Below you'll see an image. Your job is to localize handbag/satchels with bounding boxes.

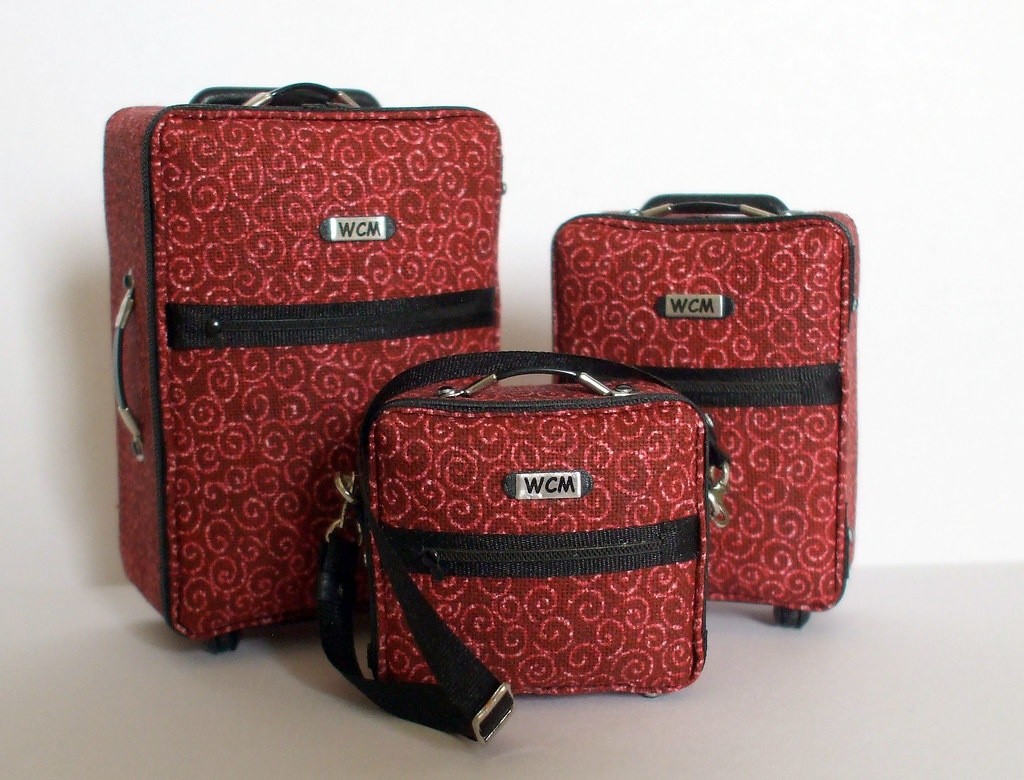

[318,351,710,741]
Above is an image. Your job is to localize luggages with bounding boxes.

[551,194,861,629]
[103,83,507,654]
